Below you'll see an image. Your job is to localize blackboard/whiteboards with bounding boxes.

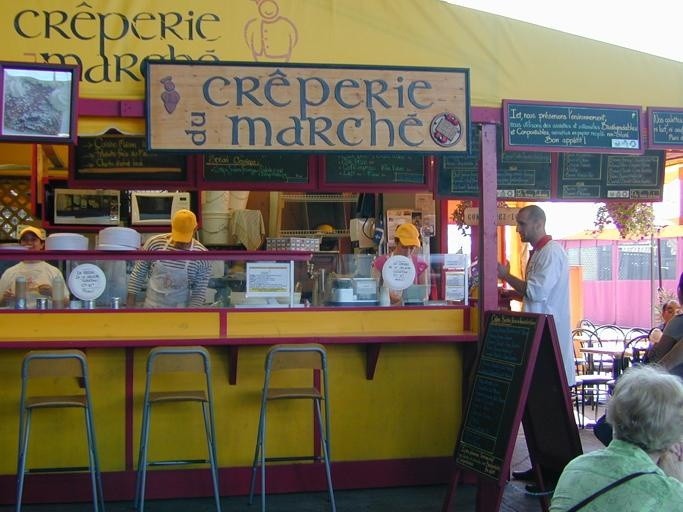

[507,101,646,155]
[647,105,683,150]
[198,131,316,190]
[70,134,197,188]
[443,126,552,201]
[452,309,546,486]
[319,133,433,191]
[555,149,664,202]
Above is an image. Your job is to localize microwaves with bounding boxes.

[52,188,120,225]
[130,191,191,226]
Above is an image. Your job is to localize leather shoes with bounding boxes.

[512,468,534,480]
[525,484,537,492]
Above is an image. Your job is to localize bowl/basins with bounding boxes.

[275,291,301,304]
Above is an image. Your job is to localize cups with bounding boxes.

[35,298,47,310]
[84,300,95,309]
[333,288,353,302]
[69,300,81,309]
[378,286,390,307]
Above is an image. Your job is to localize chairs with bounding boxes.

[244,340,338,511]
[11,346,106,511]
[566,315,657,431]
[130,343,224,511]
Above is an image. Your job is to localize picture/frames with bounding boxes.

[0,58,80,146]
[144,58,472,157]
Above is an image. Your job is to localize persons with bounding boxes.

[648,271,682,371]
[645,300,682,363]
[547,361,683,512]
[370,222,429,305]
[498,204,577,494]
[0,227,71,306]
[126,210,212,308]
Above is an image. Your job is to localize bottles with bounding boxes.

[110,297,119,310]
[51,275,64,309]
[14,276,27,310]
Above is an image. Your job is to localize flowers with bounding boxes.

[593,199,664,245]
[450,194,511,239]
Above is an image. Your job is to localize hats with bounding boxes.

[21,226,42,241]
[394,223,421,248]
[317,224,333,234]
[172,210,197,243]
